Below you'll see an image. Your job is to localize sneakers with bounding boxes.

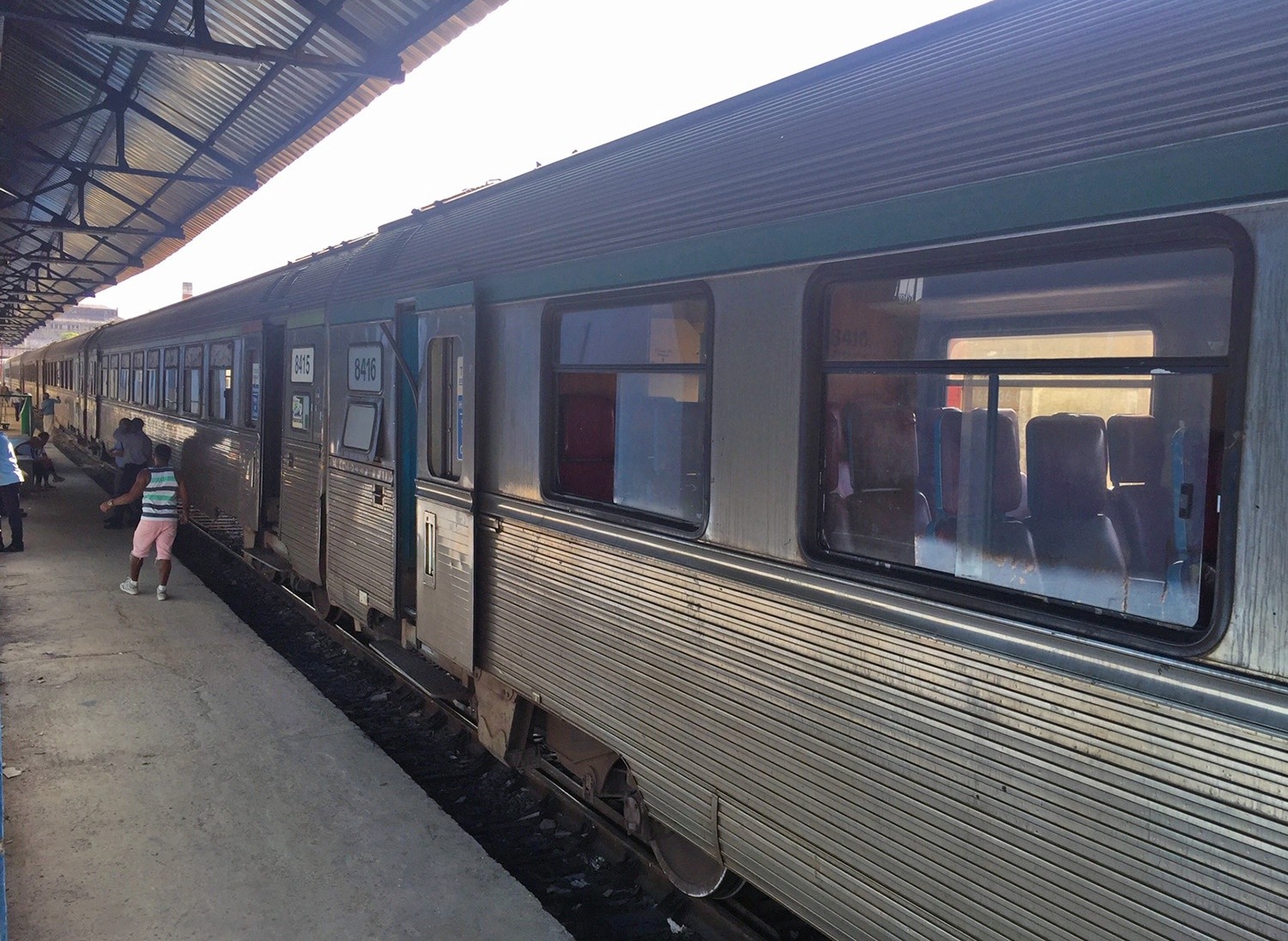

[120,576,138,594]
[156,585,167,600]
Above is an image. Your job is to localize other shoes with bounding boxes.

[0,542,24,551]
[104,517,123,530]
[52,477,65,483]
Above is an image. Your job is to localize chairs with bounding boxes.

[825,391,1195,623]
[563,387,713,510]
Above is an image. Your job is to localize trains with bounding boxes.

[0,0,1288,941]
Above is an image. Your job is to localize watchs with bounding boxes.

[107,499,114,507]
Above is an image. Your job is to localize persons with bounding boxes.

[41,393,61,433]
[99,444,190,601]
[0,382,8,407]
[104,417,154,530]
[10,388,23,421]
[0,432,65,553]
